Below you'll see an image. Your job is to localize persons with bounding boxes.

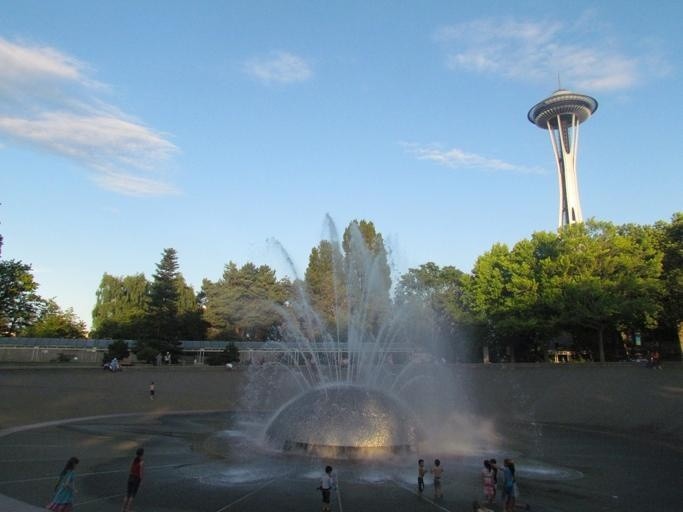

[647,348,665,371]
[163,351,172,367]
[430,458,445,504]
[46,456,80,511]
[155,352,162,367]
[118,448,144,512]
[317,465,336,511]
[108,357,119,373]
[415,459,428,502]
[148,381,157,402]
[480,458,519,511]
